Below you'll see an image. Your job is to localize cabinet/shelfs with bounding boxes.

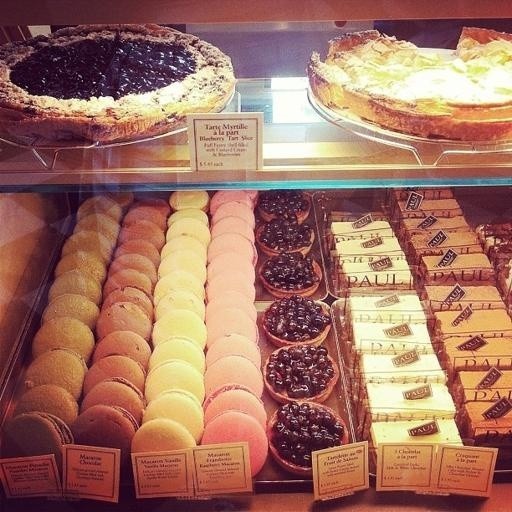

[0,0,512,510]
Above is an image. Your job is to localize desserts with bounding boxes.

[254,187,511,477]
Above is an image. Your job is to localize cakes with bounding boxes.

[0,23,236,141]
[308,26,512,141]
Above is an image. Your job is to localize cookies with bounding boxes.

[5,191,268,478]
